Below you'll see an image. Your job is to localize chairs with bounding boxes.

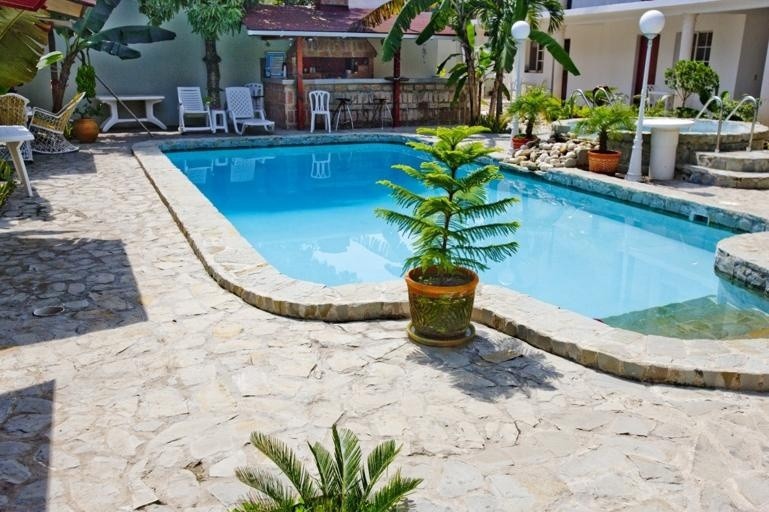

[243,83,266,120]
[224,86,275,135]
[0,92,33,161]
[29,90,85,155]
[176,86,213,135]
[307,90,331,133]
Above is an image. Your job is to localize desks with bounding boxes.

[96,93,167,131]
[0,123,34,200]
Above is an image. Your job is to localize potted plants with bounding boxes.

[369,118,521,349]
[573,100,637,175]
[503,86,558,152]
[72,105,99,144]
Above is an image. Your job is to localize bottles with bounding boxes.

[281,63,287,79]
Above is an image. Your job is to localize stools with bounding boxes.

[332,97,354,131]
[211,109,228,135]
[371,98,393,130]
